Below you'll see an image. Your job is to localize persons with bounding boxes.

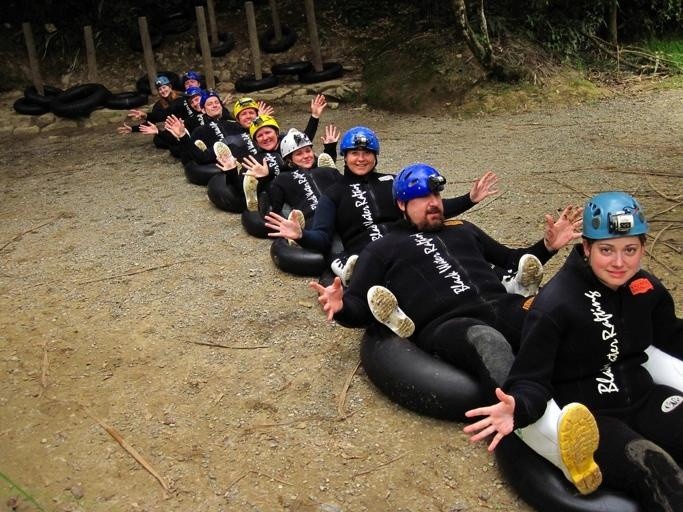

[241,128,359,288]
[215,115,340,250]
[128,76,183,123]
[164,86,265,151]
[165,101,266,165]
[164,94,339,213]
[139,87,211,152]
[463,190,683,512]
[115,70,202,138]
[264,126,544,339]
[308,164,683,496]
[164,93,275,160]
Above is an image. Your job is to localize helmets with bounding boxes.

[582,192,650,240]
[280,128,314,159]
[155,76,171,88]
[249,114,281,141]
[340,127,380,156]
[392,164,447,206]
[200,91,220,110]
[233,97,260,123]
[182,71,200,84]
[185,87,203,101]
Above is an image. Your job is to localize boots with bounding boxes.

[286,209,305,247]
[501,254,543,298]
[645,345,683,391]
[243,170,259,211]
[514,397,604,496]
[366,284,415,338]
[213,141,241,174]
[317,153,336,168]
[195,140,207,152]
[330,254,360,288]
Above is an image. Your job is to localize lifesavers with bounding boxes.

[492,426,657,512]
[104,91,148,110]
[168,142,183,156]
[240,192,282,239]
[270,238,326,277]
[186,159,223,186]
[23,85,63,105]
[316,264,346,308]
[235,72,277,93]
[14,97,51,116]
[195,30,235,56]
[259,24,297,53]
[207,173,246,214]
[271,61,312,76]
[153,135,170,149]
[129,27,164,51]
[298,62,344,83]
[136,71,179,95]
[52,83,111,118]
[180,150,193,165]
[176,74,218,92]
[359,323,499,424]
